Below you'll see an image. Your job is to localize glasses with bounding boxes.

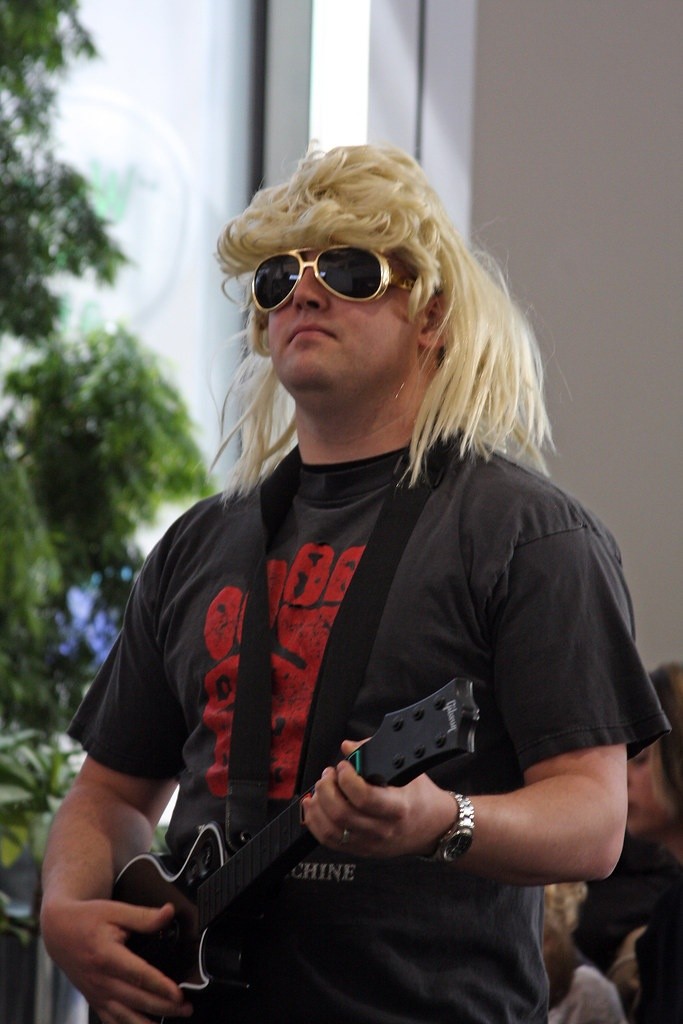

[251,244,416,313]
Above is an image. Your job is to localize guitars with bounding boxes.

[112,676,480,1008]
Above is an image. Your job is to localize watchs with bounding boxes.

[419,791,474,869]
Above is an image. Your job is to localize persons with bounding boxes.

[541,881,623,1024]
[626,661,683,914]
[38,143,662,1024]
